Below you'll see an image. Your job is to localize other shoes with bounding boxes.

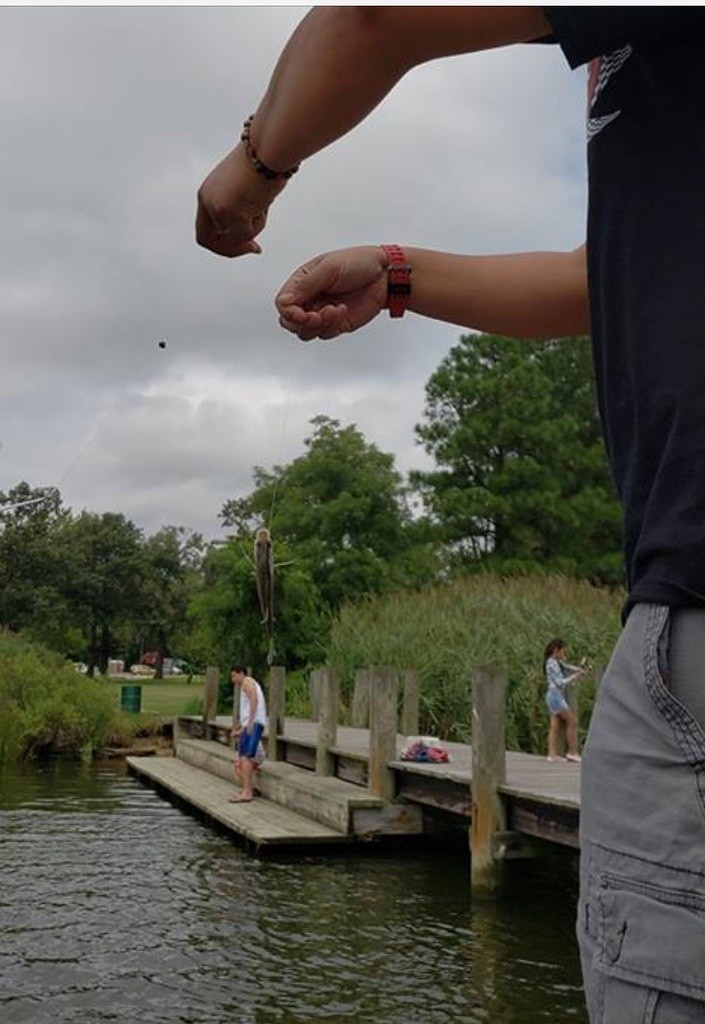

[567,754,581,762]
[547,756,568,762]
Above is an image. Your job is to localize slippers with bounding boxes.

[230,800,250,802]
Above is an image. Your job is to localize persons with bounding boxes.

[229,665,266,803]
[195,6,705,1024]
[543,639,584,763]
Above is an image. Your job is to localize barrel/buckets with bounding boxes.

[121,685,141,713]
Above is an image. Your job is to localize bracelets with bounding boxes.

[381,243,412,319]
[240,114,301,181]
